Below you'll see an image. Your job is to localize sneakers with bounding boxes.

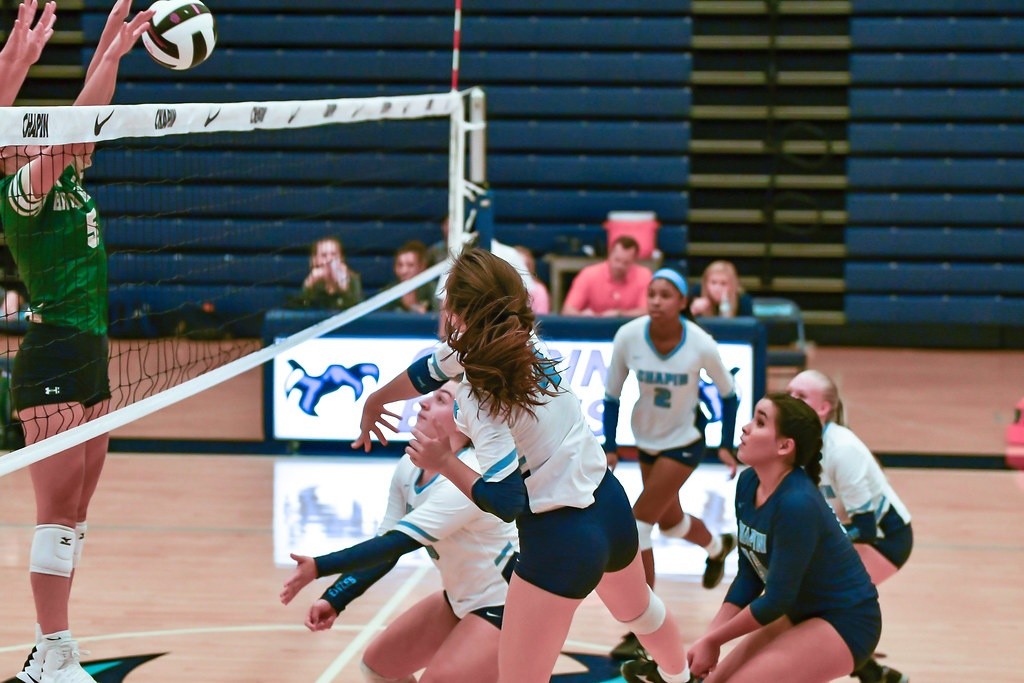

[879,666,909,682]
[703,533,736,588]
[40,641,98,683]
[15,637,64,683]
[611,631,643,660]
[621,659,697,683]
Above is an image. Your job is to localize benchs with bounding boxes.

[0,0,1024,345]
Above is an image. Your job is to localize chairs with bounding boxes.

[750,297,807,377]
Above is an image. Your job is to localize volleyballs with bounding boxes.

[141,0,218,71]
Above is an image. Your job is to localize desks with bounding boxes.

[258,308,765,466]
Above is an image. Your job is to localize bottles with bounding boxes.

[719,288,732,318]
[330,261,350,284]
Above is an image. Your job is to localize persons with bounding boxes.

[281,216,913,683]
[0,0,157,683]
